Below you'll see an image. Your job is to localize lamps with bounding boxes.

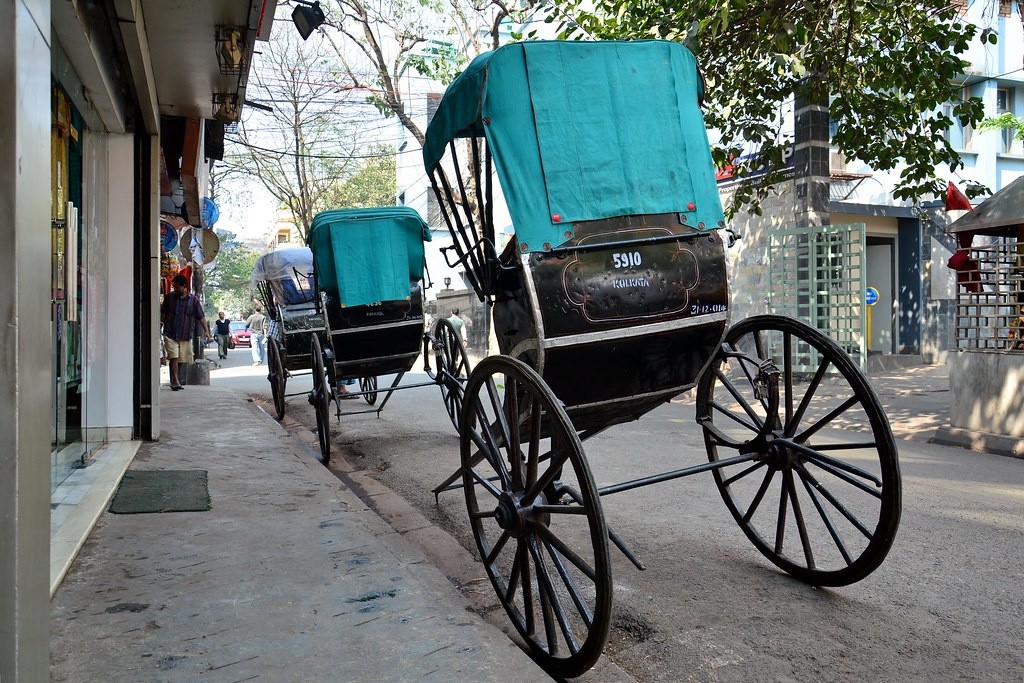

[214,97,235,126]
[221,29,241,71]
[291,0,325,40]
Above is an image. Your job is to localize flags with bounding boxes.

[946,182,975,216]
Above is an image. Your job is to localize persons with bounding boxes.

[337,378,360,399]
[215,311,230,360]
[159,275,211,391]
[244,306,268,366]
[444,308,468,366]
[419,308,436,355]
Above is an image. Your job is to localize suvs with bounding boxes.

[214,321,252,349]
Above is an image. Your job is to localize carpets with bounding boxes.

[109,470,212,514]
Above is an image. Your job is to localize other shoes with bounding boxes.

[220,356,223,359]
[252,361,261,366]
[223,357,226,359]
[170,383,184,391]
[341,391,360,399]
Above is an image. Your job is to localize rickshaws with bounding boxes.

[308,207,472,463]
[432,40,903,678]
[252,247,328,419]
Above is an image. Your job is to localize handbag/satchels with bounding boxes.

[227,337,235,349]
